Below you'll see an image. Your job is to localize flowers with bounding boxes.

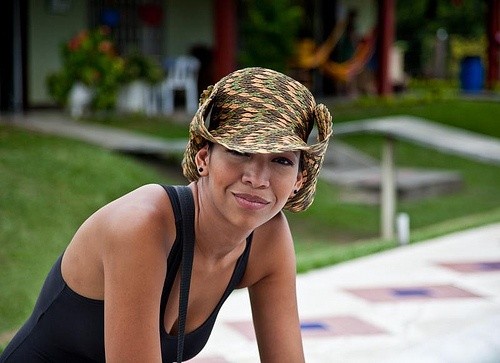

[42,26,164,92]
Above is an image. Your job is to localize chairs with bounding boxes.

[150,57,199,119]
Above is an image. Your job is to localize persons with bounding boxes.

[0,67,333,363]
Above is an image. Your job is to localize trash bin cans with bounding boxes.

[461,54,486,93]
